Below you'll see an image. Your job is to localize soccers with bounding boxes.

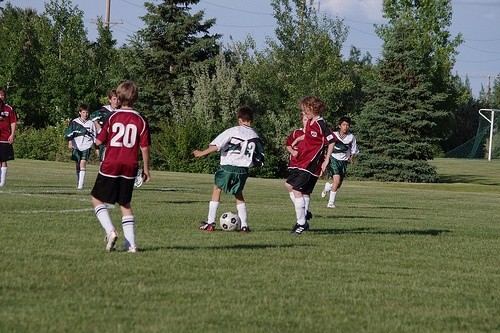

[219,212,240,232]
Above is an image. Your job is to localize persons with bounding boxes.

[65,103,101,190]
[0,86,18,187]
[90,80,151,253]
[89,89,120,165]
[192,106,265,234]
[321,116,359,208]
[285,110,313,221]
[285,95,338,234]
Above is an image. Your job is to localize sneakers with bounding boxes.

[235,226,249,231]
[327,203,336,208]
[305,210,312,220]
[104,230,118,252]
[198,221,216,231]
[290,220,309,235]
[322,181,331,198]
[118,246,138,252]
